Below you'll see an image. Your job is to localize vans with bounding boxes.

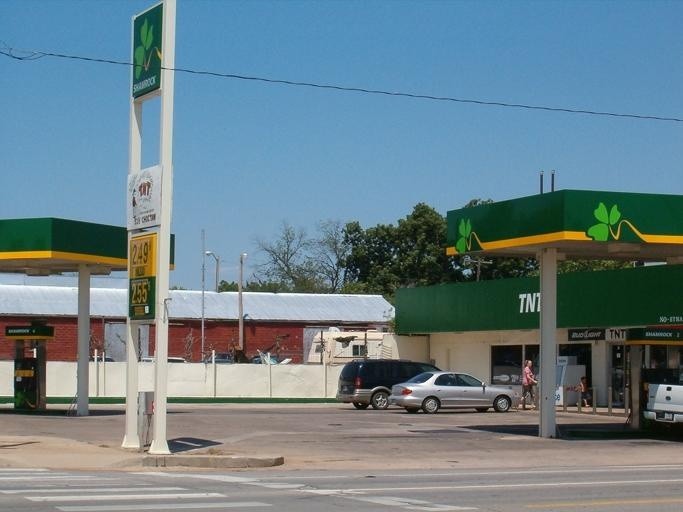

[336,360,442,410]
[307,331,395,365]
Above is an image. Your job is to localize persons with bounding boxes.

[515,360,538,411]
[574,376,591,407]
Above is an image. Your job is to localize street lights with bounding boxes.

[205,250,253,350]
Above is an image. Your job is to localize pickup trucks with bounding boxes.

[643,383,683,424]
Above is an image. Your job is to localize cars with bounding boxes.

[387,370,520,412]
[86,353,280,364]
